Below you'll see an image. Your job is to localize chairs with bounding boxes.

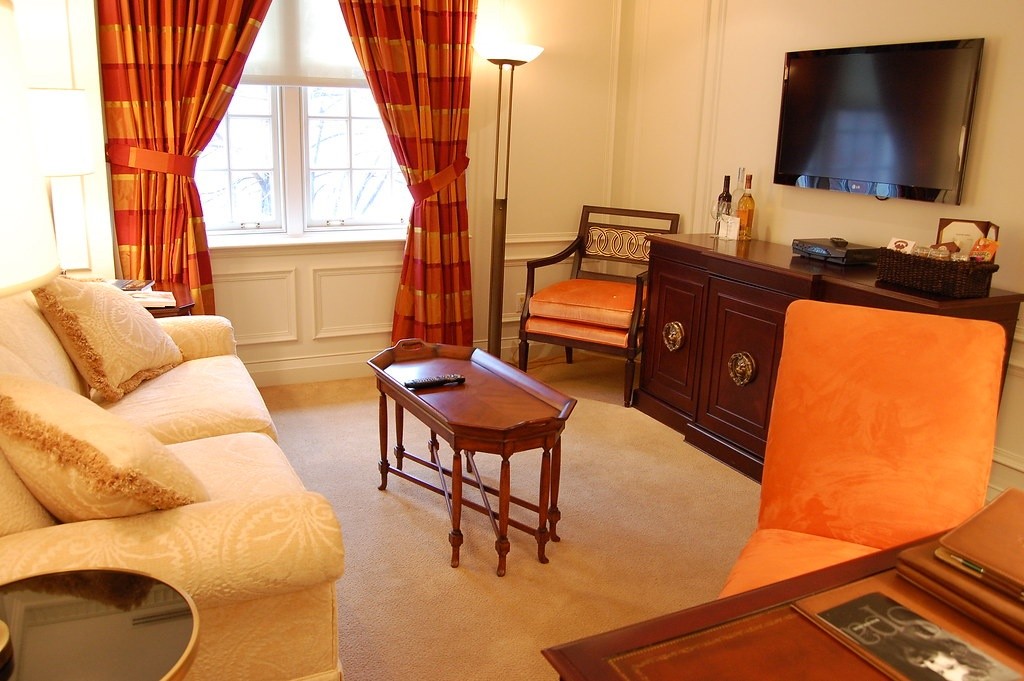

[518,203,680,409]
[716,296,1008,598]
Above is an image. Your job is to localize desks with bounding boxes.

[364,337,579,577]
[541,521,1022,681]
[0,567,200,681]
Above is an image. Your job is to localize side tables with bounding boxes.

[103,279,196,318]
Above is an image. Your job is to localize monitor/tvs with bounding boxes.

[773,38,984,205]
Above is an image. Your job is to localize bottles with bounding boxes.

[737,174,755,241]
[715,175,732,235]
[731,167,745,218]
[911,246,970,262]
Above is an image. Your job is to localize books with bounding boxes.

[895,489,1024,649]
[112,280,177,306]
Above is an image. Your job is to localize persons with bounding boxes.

[921,639,994,681]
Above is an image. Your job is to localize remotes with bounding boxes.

[830,238,848,247]
[404,374,465,388]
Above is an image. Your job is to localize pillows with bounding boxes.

[32,275,186,403]
[0,370,211,524]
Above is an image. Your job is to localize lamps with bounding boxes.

[475,38,546,365]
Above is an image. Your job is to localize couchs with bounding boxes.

[0,264,346,681]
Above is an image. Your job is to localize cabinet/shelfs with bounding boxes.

[631,237,1024,489]
